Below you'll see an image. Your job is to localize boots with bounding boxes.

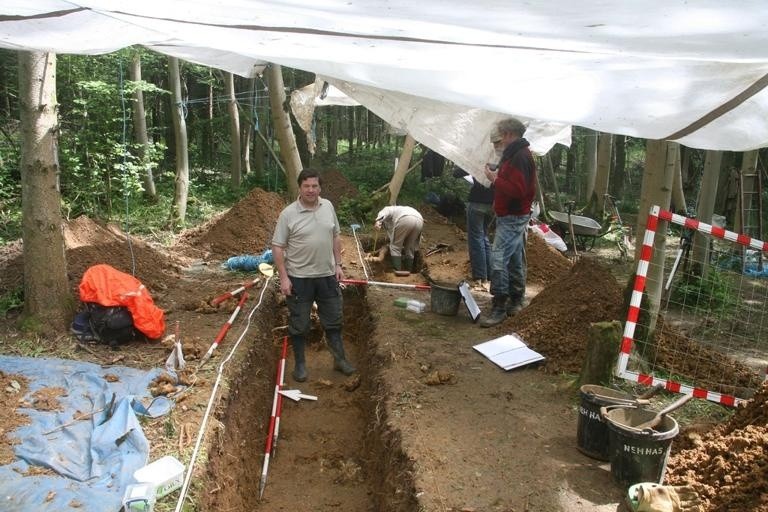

[392,256,403,271]
[404,259,416,272]
[293,336,308,382]
[325,331,360,376]
[507,292,522,316]
[480,296,507,327]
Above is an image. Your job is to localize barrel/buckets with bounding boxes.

[429,281,461,317]
[600,403,680,491]
[575,383,640,462]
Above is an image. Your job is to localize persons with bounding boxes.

[375,205,424,273]
[479,118,538,329]
[451,168,494,281]
[489,126,536,298]
[272,168,357,381]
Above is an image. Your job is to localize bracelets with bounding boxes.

[335,261,342,266]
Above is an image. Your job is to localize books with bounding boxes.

[472,334,545,370]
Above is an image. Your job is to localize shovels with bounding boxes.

[424,244,450,257]
[660,236,690,312]
[278,390,317,403]
[634,394,694,430]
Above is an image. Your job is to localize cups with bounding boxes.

[487,158,496,171]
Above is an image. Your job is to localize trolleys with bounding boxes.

[548,192,632,251]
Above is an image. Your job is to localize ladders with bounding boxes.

[739,170,763,275]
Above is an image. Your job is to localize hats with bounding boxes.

[489,126,504,142]
